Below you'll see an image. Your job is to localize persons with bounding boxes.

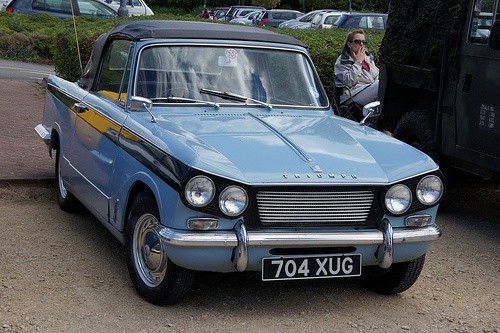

[334,29,379,106]
[202,9,210,18]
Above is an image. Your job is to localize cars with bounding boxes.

[78,0,156,18]
[195,5,266,26]
[311,10,355,32]
[331,12,388,46]
[279,9,328,31]
[6,0,123,22]
[228,10,262,26]
[252,8,306,29]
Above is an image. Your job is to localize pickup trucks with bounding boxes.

[33,18,448,307]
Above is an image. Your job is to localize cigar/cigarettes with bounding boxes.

[361,46,363,50]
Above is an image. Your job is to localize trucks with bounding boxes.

[375,0,500,187]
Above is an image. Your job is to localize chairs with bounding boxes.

[333,77,362,123]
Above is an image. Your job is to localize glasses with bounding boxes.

[348,39,367,45]
